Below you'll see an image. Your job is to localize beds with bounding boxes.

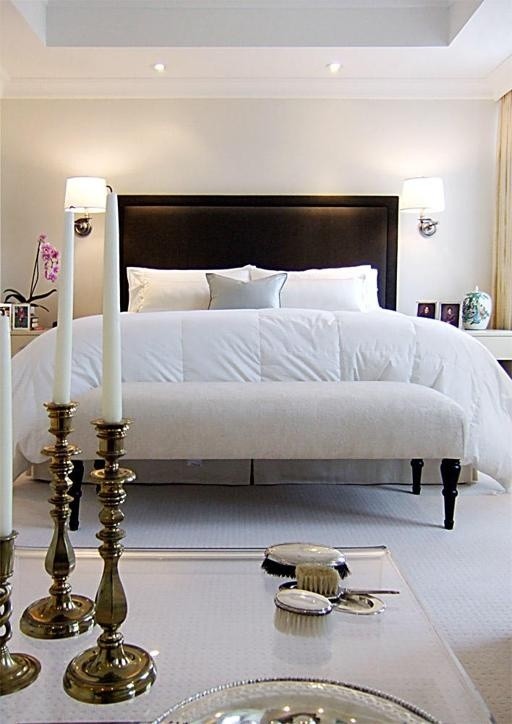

[10,191,511,530]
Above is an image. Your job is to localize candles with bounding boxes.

[0,309,14,539]
[52,202,82,405]
[97,182,125,426]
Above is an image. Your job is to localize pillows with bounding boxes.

[124,261,258,315]
[203,268,288,311]
[247,263,379,313]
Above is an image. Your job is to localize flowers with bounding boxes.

[1,229,61,307]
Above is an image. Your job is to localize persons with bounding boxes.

[17,307,26,327]
[446,307,457,325]
[420,306,432,318]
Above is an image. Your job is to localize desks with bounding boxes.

[0,541,498,724]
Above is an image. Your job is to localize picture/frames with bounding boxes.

[12,301,30,331]
[415,296,439,322]
[0,301,13,330]
[437,298,461,329]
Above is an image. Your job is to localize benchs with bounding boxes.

[16,373,473,537]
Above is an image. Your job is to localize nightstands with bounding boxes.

[455,327,512,367]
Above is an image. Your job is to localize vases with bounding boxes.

[27,304,40,329]
[459,282,493,330]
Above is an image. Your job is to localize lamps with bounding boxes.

[396,175,447,235]
[60,175,114,237]
